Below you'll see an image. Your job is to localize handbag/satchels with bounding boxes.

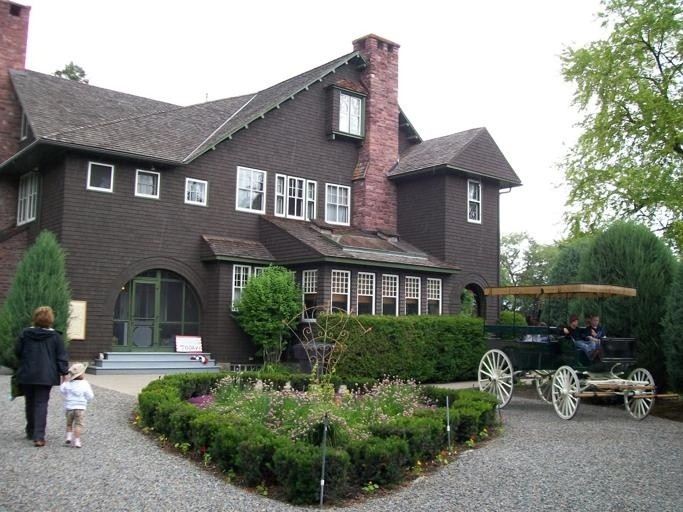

[11,374,25,397]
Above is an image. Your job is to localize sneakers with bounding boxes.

[64,437,72,444]
[72,439,81,447]
[34,440,45,446]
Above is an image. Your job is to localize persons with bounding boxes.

[537,320,553,344]
[521,314,539,342]
[13,304,68,447]
[59,361,94,450]
[556,314,601,363]
[586,312,607,348]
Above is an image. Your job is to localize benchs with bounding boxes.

[557,325,600,367]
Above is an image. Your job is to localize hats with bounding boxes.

[68,362,89,381]
[570,314,578,323]
